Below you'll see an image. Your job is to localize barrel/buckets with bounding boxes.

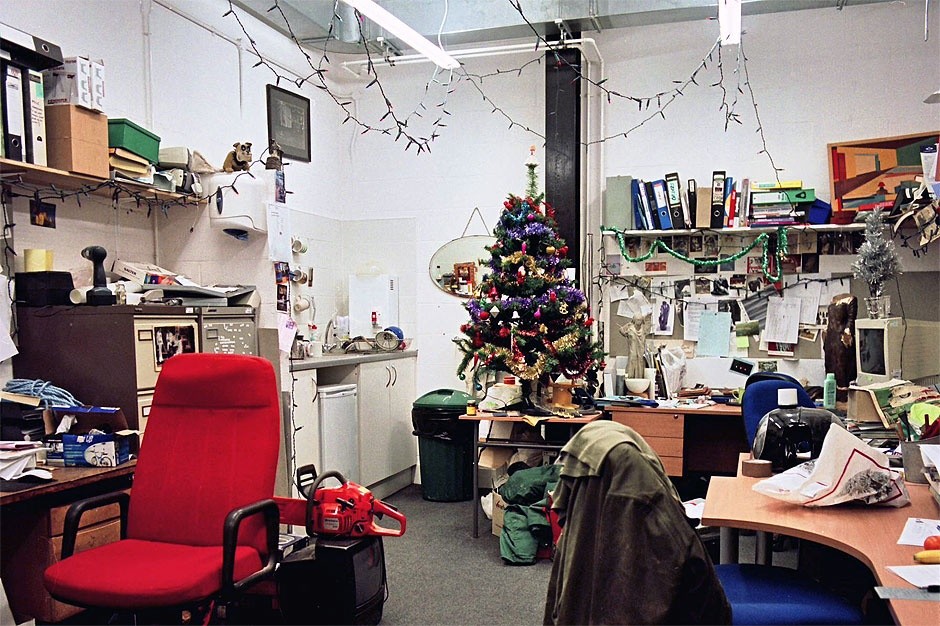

[900,435,939,484]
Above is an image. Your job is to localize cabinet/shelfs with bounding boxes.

[324,355,419,490]
[283,367,321,541]
[0,488,139,626]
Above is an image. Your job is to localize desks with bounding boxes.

[460,407,602,549]
[603,396,847,482]
[0,452,140,626]
[700,473,940,626]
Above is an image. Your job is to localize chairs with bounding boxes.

[748,406,846,474]
[553,413,865,626]
[745,371,803,386]
[44,352,285,626]
[740,377,821,449]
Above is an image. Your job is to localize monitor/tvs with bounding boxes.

[854,318,940,387]
[275,535,386,626]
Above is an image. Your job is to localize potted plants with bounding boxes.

[849,205,902,321]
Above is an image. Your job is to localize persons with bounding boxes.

[705,237,717,256]
[619,314,648,379]
[660,301,669,330]
[627,239,639,258]
[674,237,688,257]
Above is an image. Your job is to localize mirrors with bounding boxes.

[429,234,499,298]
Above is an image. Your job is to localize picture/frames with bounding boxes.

[266,82,312,164]
[826,129,940,214]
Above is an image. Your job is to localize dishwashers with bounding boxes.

[317,383,360,490]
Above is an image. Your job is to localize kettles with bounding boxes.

[289,336,305,360]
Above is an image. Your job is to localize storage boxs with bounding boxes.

[113,258,177,286]
[477,449,510,490]
[846,391,881,420]
[798,198,829,223]
[40,409,64,463]
[89,59,107,112]
[605,175,633,230]
[48,140,110,180]
[45,54,91,109]
[491,489,508,537]
[488,420,518,440]
[45,105,109,145]
[695,187,712,228]
[64,402,136,472]
[108,117,161,163]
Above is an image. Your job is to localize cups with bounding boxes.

[307,341,322,357]
[292,265,308,284]
[294,294,311,311]
[291,236,307,253]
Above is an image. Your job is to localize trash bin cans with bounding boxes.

[412,389,481,501]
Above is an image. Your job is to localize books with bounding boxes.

[723,176,804,227]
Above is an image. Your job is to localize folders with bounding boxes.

[651,179,673,229]
[711,171,725,228]
[638,183,654,230]
[749,187,815,202]
[665,173,684,229]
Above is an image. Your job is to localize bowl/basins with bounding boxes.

[399,338,412,350]
[375,329,400,349]
[625,378,650,393]
[354,341,374,351]
[384,326,403,342]
[742,459,772,477]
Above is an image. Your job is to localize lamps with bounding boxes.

[338,0,462,69]
[718,0,742,47]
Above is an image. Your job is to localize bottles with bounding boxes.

[466,400,477,416]
[823,373,836,409]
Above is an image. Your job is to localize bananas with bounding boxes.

[913,550,940,564]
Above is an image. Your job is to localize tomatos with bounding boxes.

[924,535,940,550]
